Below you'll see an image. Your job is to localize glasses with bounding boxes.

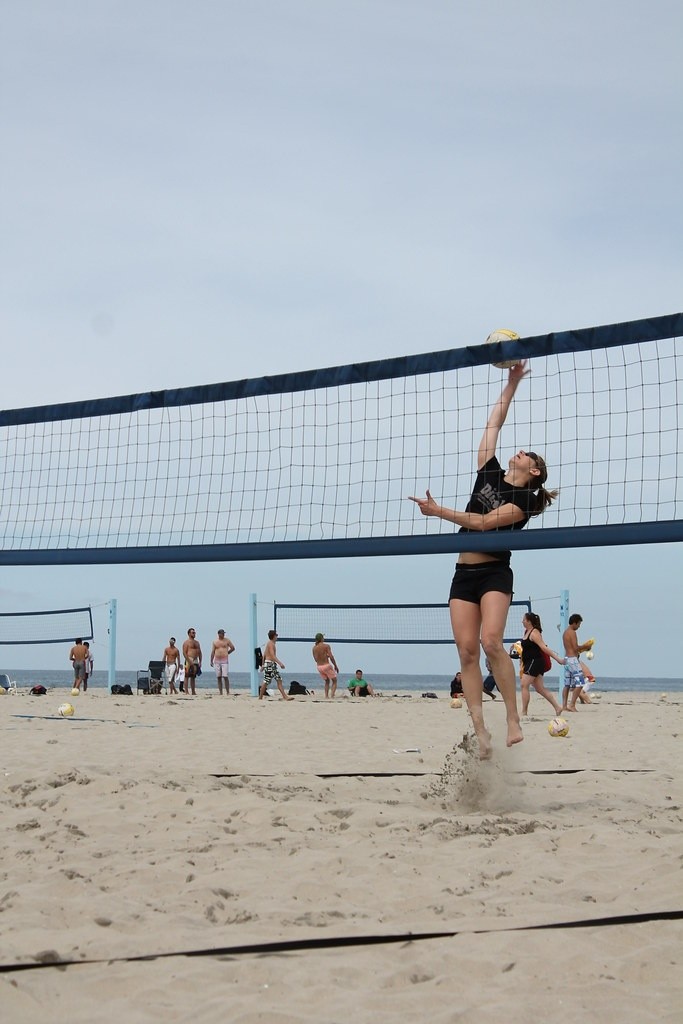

[526,452,539,470]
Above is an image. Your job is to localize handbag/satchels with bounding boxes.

[541,644,551,672]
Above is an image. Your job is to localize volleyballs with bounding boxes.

[0,688,4,694]
[58,703,74,716]
[589,693,602,699]
[71,688,79,696]
[548,718,570,737]
[662,694,667,698]
[586,652,594,660]
[450,699,462,708]
[486,327,523,369]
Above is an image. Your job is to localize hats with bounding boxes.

[218,629,226,633]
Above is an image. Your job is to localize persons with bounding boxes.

[450,672,465,698]
[313,633,339,698]
[511,612,567,716]
[483,658,500,700]
[163,638,180,694]
[562,613,595,711]
[259,630,295,700]
[183,628,202,695]
[210,629,235,695]
[178,664,185,692]
[409,360,558,760]
[70,638,94,691]
[347,670,376,697]
